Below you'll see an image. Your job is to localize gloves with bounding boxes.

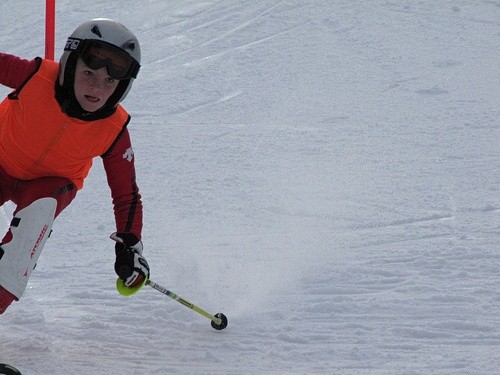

[110,231,150,289]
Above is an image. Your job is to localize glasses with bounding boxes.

[64,37,141,79]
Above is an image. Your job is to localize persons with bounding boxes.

[1,16,151,375]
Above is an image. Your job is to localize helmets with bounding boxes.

[54,17,142,121]
